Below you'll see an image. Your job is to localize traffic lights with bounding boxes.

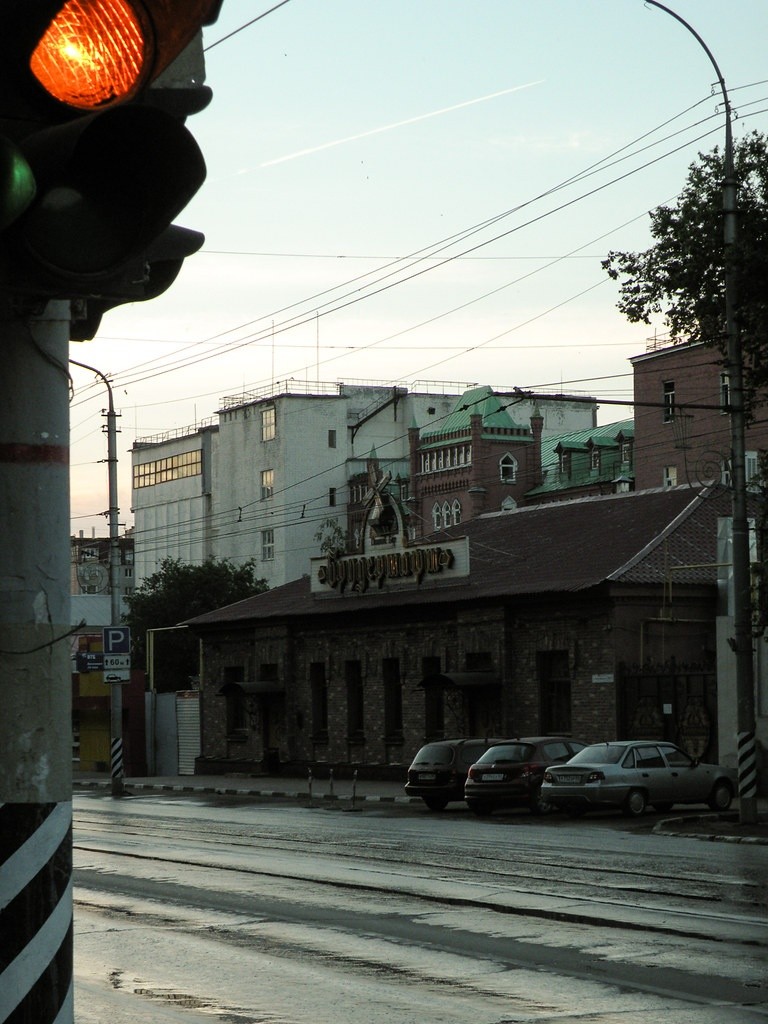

[0,1,224,297]
[73,0,224,354]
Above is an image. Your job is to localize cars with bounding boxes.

[463,736,617,818]
[540,741,738,817]
[403,736,507,813]
